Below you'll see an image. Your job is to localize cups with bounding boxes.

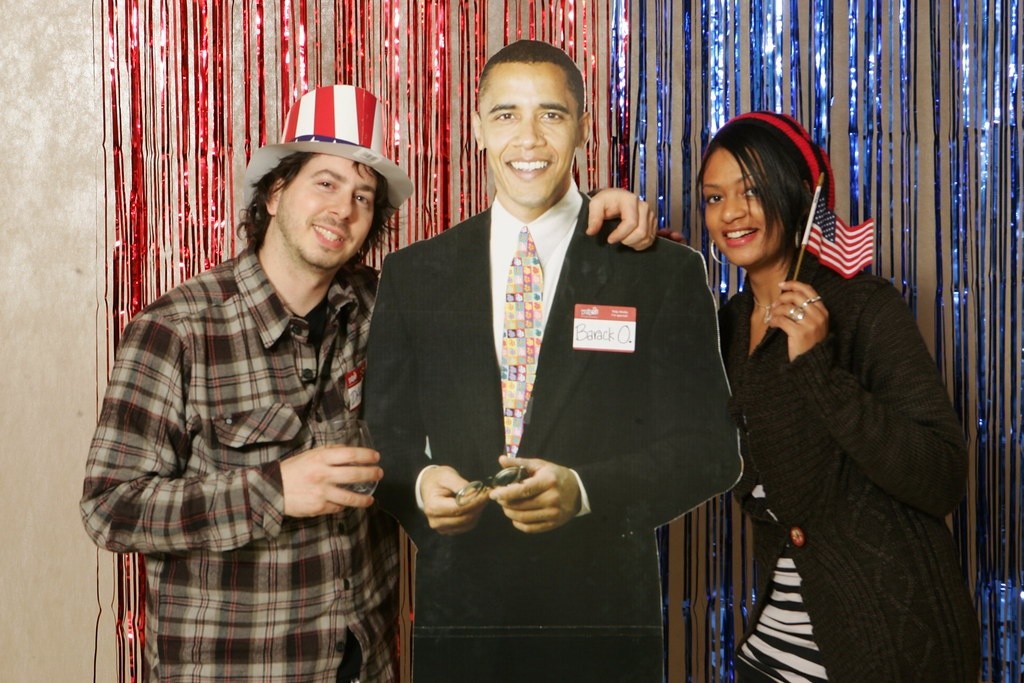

[324,419,379,496]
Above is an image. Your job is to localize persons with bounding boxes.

[658,112,983,683]
[79,84,656,683]
[360,38,745,682]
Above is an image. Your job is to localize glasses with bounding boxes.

[456,465,524,507]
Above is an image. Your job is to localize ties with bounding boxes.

[501,226,545,459]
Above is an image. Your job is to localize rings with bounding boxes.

[801,298,811,308]
[789,305,804,322]
[811,295,822,303]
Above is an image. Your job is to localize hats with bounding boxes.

[245,84,414,226]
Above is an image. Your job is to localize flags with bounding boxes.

[805,194,874,279]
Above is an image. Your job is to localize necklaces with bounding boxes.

[753,296,778,325]
